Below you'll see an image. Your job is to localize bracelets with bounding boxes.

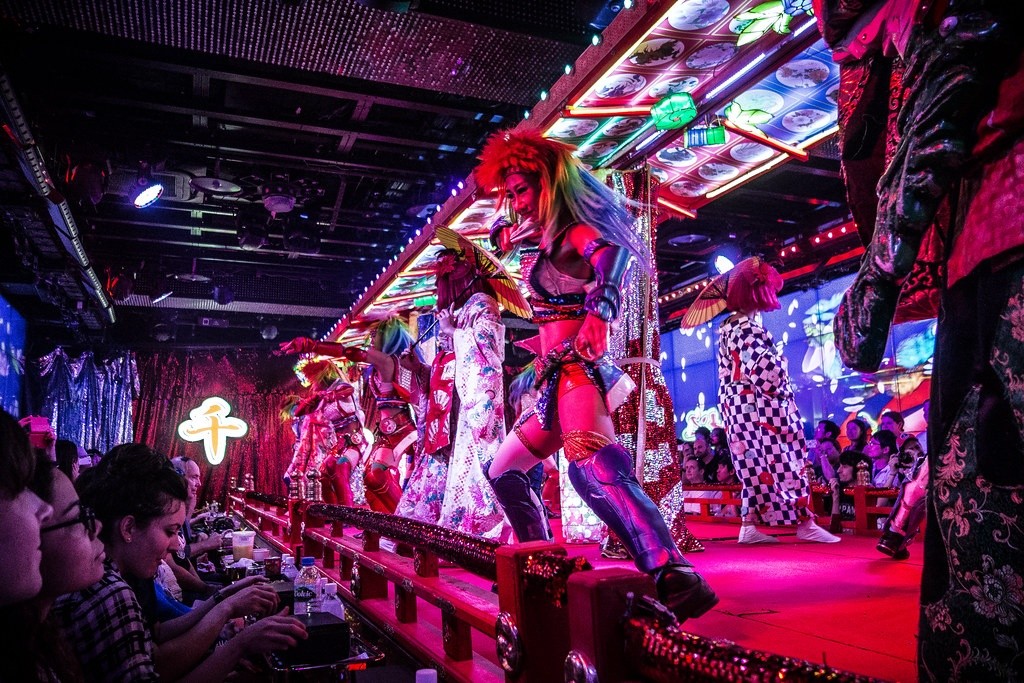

[213,591,224,603]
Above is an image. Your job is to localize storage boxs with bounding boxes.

[277,611,352,662]
[257,581,323,621]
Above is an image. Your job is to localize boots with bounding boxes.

[568,445,718,623]
[482,466,554,592]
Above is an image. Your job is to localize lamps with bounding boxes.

[124,159,171,208]
[144,271,174,306]
[650,89,698,133]
[212,282,233,305]
[712,245,743,274]
[683,118,724,149]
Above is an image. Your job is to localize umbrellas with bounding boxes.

[680,256,759,329]
[401,224,533,358]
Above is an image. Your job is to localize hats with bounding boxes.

[897,432,918,452]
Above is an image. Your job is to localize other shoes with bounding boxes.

[877,533,910,559]
[737,526,777,544]
[796,521,841,543]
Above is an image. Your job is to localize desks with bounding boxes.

[189,512,449,683]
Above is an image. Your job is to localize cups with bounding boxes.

[230,531,256,563]
[245,566,266,579]
[253,550,269,563]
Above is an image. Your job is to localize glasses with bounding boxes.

[869,441,881,446]
[40,506,97,533]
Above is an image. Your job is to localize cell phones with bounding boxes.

[678,445,684,451]
[805,440,817,448]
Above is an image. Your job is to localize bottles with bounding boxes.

[279,554,291,575]
[284,557,298,582]
[293,556,322,618]
[319,578,344,620]
[416,669,437,683]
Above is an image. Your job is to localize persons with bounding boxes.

[676,425,743,518]
[716,260,842,543]
[808,398,930,558]
[0,403,310,683]
[281,129,722,628]
[808,0,1023,683]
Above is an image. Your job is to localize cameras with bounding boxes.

[893,452,912,469]
